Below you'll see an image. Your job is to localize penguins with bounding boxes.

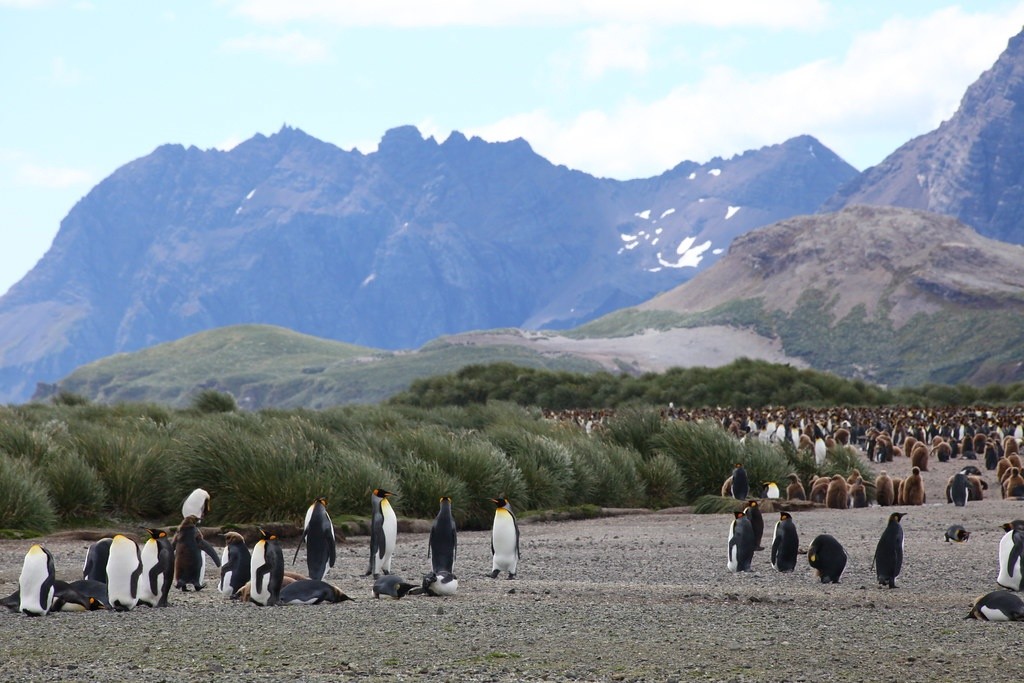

[726,511,755,574]
[742,500,765,551]
[371,574,418,601]
[170,514,222,593]
[49,578,112,614]
[944,525,971,543]
[962,591,1024,622]
[82,537,115,607]
[174,487,210,528]
[870,512,908,589]
[1,543,55,617]
[134,526,175,608]
[426,496,458,578]
[769,511,799,573]
[275,579,357,606]
[538,401,1024,509]
[485,497,521,580]
[409,571,459,597]
[250,526,284,608]
[807,534,848,585]
[996,519,1024,591]
[105,534,144,613]
[218,531,252,600]
[363,489,398,583]
[292,496,336,582]
